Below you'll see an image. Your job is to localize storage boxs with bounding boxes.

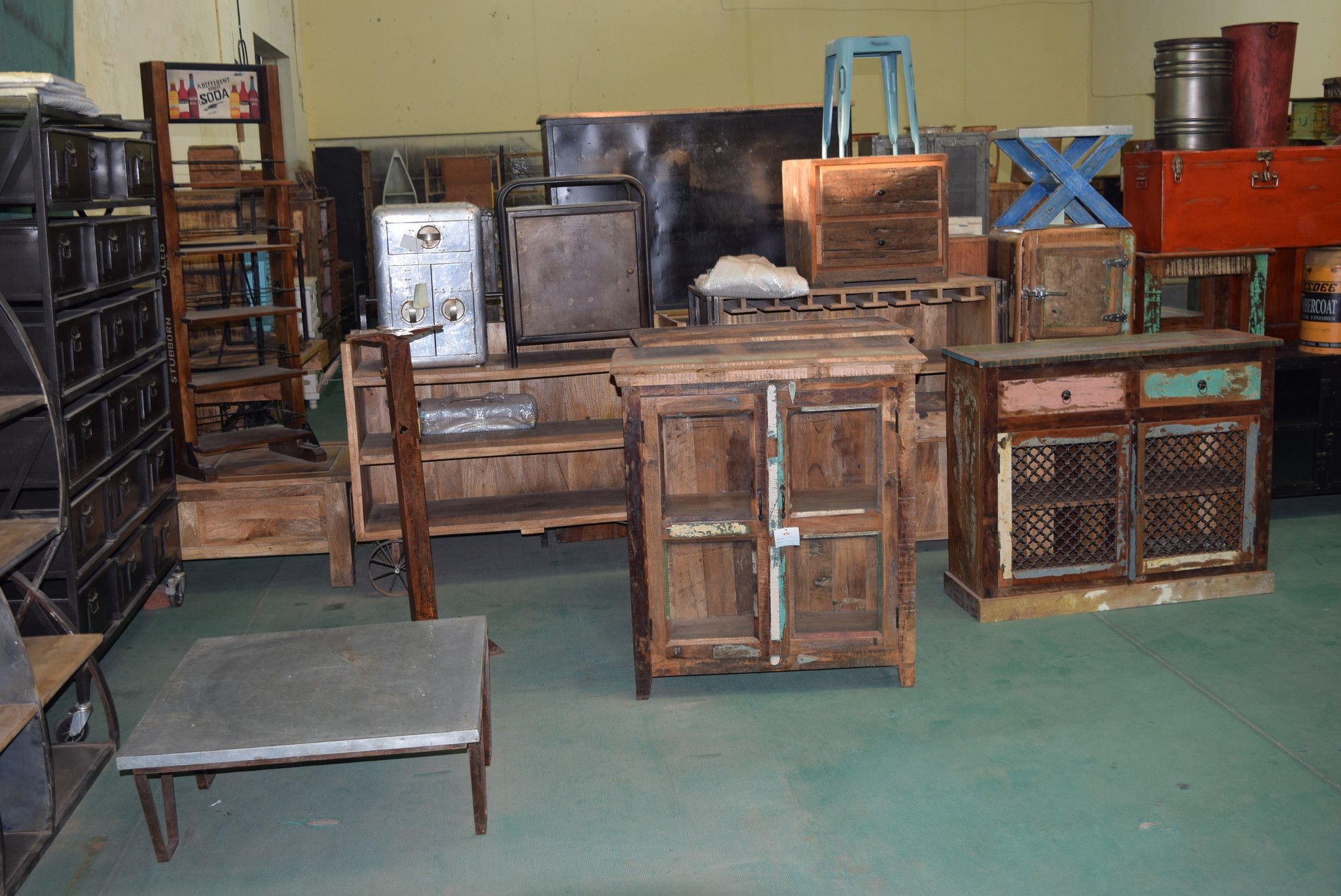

[1121,145,1341,256]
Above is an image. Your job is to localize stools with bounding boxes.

[989,124,1133,229]
[821,35,920,160]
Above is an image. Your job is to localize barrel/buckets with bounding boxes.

[1152,37,1235,150]
[1221,21,1300,147]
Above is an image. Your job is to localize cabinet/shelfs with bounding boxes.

[0,49,1278,896]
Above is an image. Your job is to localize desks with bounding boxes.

[116,616,494,865]
